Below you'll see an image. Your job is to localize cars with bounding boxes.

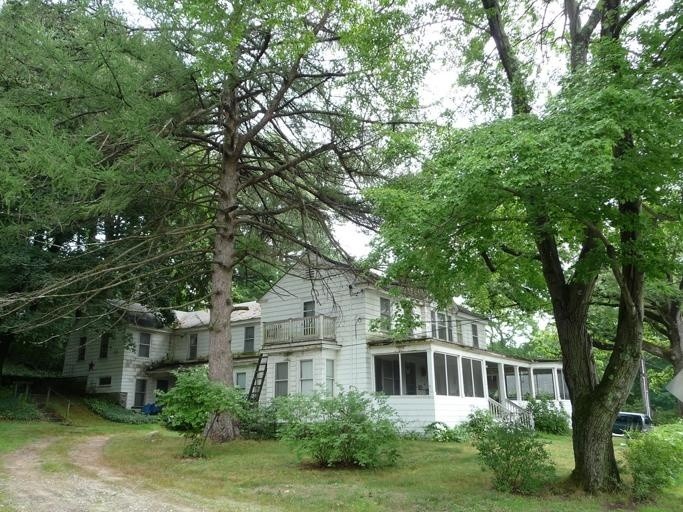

[611,412,654,437]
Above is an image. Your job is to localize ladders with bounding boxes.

[247,354,268,402]
